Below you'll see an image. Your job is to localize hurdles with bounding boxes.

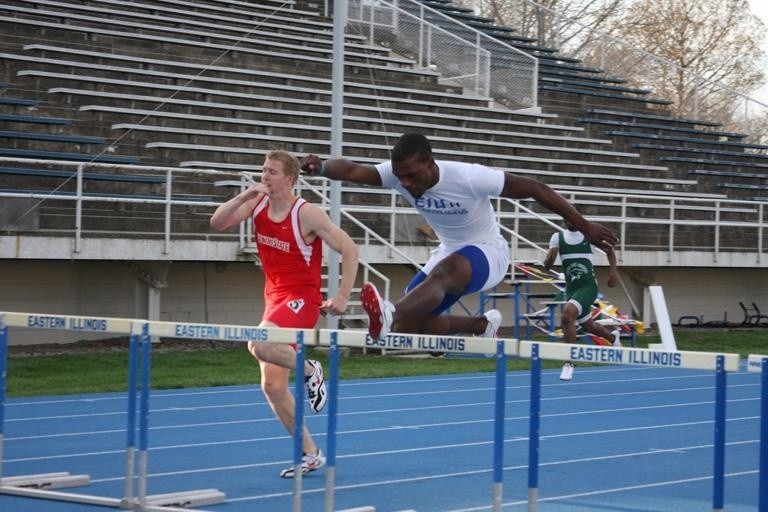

[0,312,767,511]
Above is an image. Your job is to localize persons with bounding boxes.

[538,205,622,380]
[210,150,359,478]
[298,133,619,357]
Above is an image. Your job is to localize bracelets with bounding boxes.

[319,162,325,177]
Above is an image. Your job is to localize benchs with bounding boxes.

[0,0,768,251]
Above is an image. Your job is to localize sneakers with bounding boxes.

[305,359,327,413]
[611,329,622,347]
[560,365,575,381]
[280,447,327,479]
[473,309,502,358]
[361,283,395,339]
